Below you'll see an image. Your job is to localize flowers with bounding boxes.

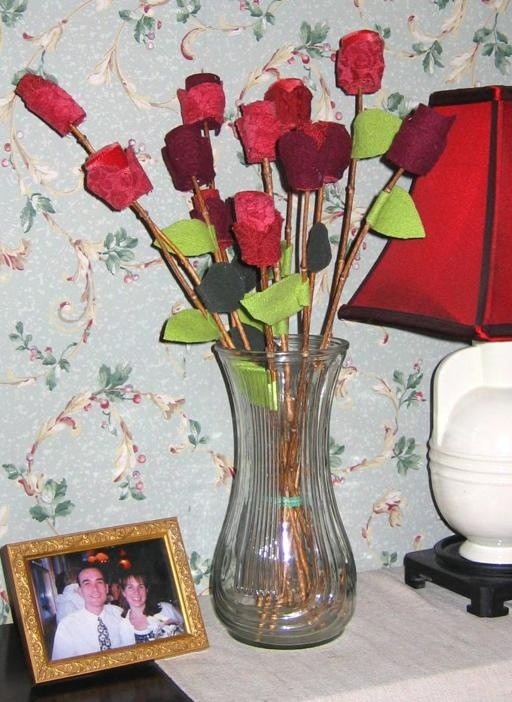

[11,31,461,638]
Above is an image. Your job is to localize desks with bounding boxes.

[2,562,510,701]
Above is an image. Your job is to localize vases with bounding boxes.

[210,334,357,649]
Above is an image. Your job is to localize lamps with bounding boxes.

[335,83,512,619]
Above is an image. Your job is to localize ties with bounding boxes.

[95,616,112,651]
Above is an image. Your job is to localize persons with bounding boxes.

[115,568,184,644]
[52,561,136,664]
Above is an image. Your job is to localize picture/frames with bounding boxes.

[1,516,210,684]
